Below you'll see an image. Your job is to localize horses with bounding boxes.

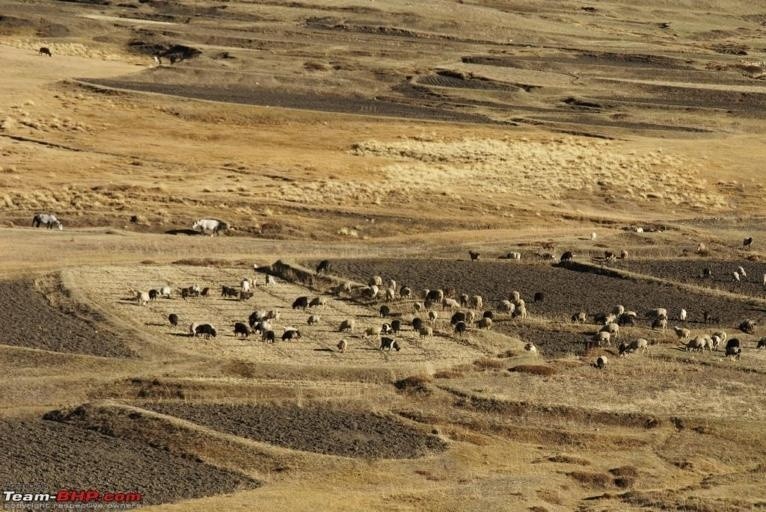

[193,219,220,237]
[31,214,63,230]
[39,48,52,57]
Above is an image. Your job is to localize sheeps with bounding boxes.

[128,275,544,353]
[571,304,766,369]
